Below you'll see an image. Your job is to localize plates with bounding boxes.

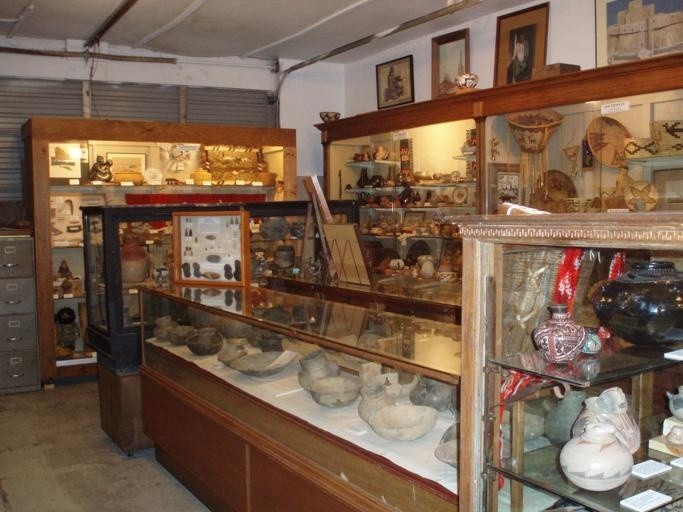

[453,185,469,204]
[623,181,659,213]
[587,117,633,168]
[528,170,577,215]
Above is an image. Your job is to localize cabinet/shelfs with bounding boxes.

[0,237,41,395]
[20,52,682,511]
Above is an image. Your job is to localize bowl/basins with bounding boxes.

[506,108,565,154]
[455,73,478,89]
[436,272,457,283]
[320,112,340,122]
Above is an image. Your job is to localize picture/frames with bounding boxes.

[374,0,550,112]
